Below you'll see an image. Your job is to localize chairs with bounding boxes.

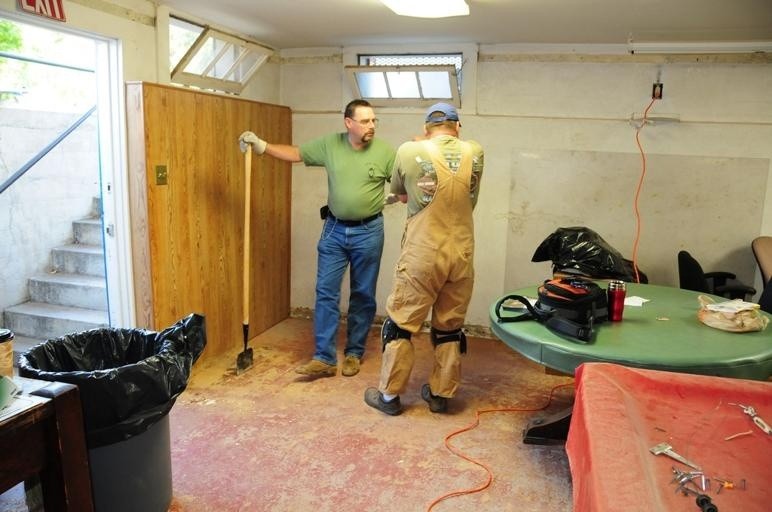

[677,251,758,303]
[744,234,772,310]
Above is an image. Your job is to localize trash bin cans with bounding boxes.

[18,328,172,512]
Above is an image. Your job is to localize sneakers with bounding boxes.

[365,387,403,416]
[294,359,338,377]
[341,355,362,377]
[421,383,450,413]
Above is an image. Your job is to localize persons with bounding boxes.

[238,100,401,378]
[364,102,485,415]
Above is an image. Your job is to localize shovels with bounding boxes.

[236,143,254,376]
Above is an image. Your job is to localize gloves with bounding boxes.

[238,130,269,155]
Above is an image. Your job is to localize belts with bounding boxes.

[328,209,382,227]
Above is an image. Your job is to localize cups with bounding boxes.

[607,280,626,322]
[1,330,15,381]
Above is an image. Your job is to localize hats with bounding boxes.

[424,103,462,127]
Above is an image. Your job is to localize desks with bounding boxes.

[1,371,101,511]
[559,354,770,512]
[486,277,770,448]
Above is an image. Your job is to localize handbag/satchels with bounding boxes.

[495,275,613,343]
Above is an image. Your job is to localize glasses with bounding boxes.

[352,118,378,125]
